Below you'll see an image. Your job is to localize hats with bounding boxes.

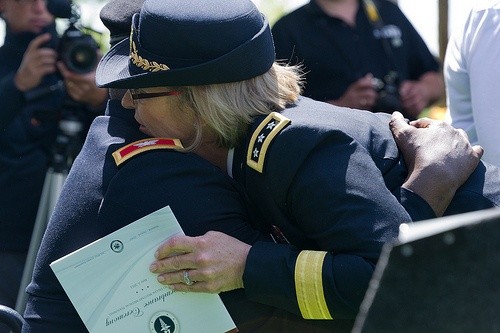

[96,0,276,89]
[100,0,144,45]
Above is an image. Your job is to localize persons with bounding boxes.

[442,0,500,166]
[0,0,111,315]
[272,0,448,121]
[20,0,486,332]
[95,0,500,332]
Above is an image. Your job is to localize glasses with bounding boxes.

[126,89,186,105]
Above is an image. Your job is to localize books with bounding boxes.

[50,205,240,333]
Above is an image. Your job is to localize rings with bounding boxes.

[183,269,195,285]
[359,98,368,108]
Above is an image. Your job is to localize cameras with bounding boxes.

[37,0,100,74]
